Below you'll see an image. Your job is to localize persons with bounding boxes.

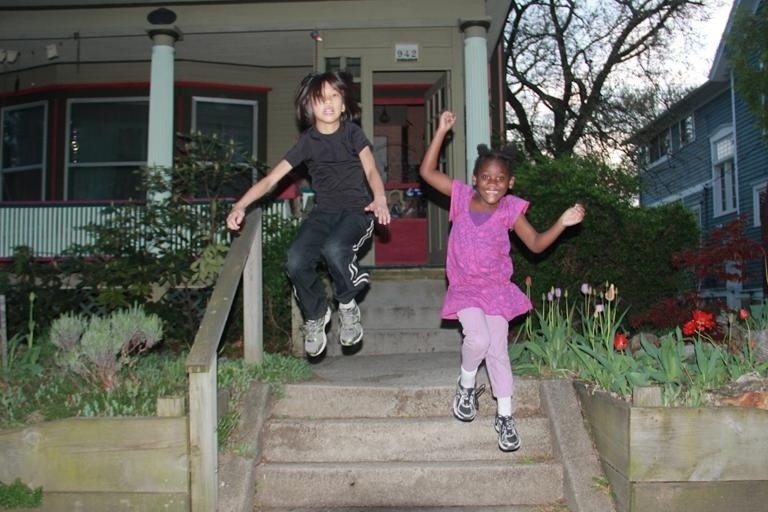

[419,107,588,452]
[224,66,390,360]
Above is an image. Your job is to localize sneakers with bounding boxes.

[452,374,478,422]
[493,413,521,451]
[302,305,333,357]
[336,299,364,347]
